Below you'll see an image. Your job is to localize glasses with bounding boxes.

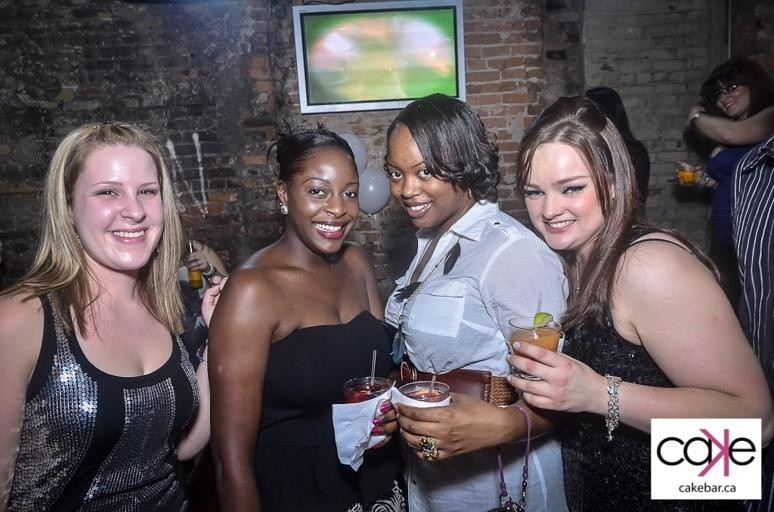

[707,84,740,100]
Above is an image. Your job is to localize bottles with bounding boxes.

[187,240,204,289]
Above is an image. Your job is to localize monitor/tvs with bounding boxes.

[292,0,466,114]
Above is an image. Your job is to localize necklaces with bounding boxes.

[390,234,463,364]
[575,261,580,297]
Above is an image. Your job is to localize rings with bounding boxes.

[417,437,440,460]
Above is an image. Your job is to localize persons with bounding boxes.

[585,87,650,219]
[506,87,774,512]
[676,55,774,315]
[189,118,409,512]
[384,93,570,512]
[0,120,230,512]
[729,136,774,512]
[177,238,229,300]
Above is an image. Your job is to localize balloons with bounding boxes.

[358,167,391,216]
[337,132,367,176]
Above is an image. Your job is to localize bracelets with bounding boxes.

[196,338,208,365]
[697,172,710,189]
[604,373,622,442]
[202,261,215,279]
[689,109,706,129]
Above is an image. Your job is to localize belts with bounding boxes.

[393,365,516,411]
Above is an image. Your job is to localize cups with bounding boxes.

[397,381,452,402]
[343,377,392,404]
[507,316,562,382]
[676,159,703,187]
